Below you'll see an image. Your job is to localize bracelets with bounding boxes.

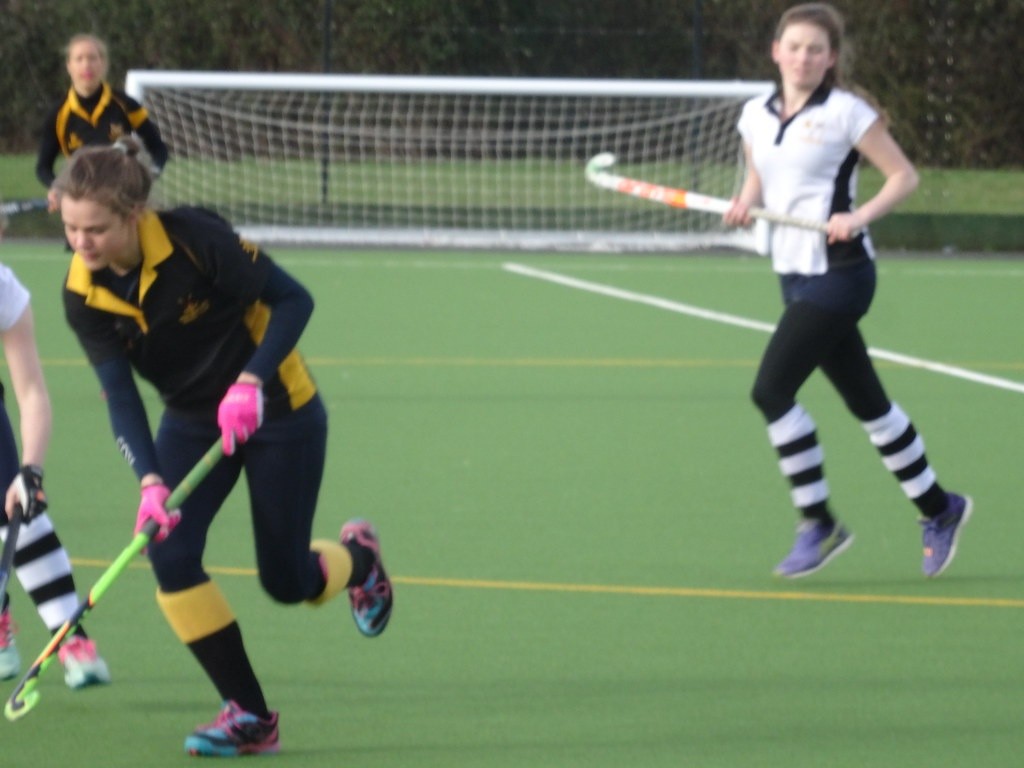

[873,197,888,216]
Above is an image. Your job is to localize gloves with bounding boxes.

[133,483,182,557]
[217,383,264,458]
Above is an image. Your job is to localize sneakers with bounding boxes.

[0,593,21,680]
[773,517,855,578]
[184,699,281,758]
[339,517,393,639]
[58,635,111,690]
[917,492,973,578]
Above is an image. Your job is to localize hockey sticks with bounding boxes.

[584,150,861,240]
[2,431,224,723]
[1,500,25,615]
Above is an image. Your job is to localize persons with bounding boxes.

[52,138,393,757]
[723,4,973,579]
[35,32,169,253]
[0,264,110,691]
